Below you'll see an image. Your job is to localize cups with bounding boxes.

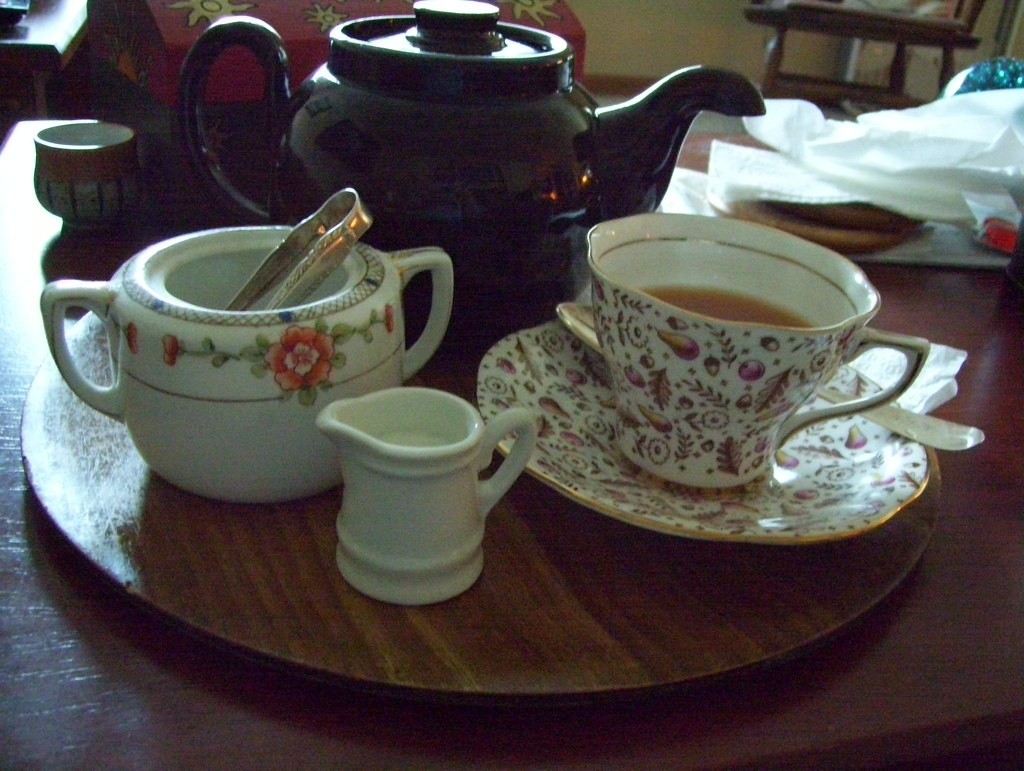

[34,120,140,228]
[41,224,453,502]
[317,387,538,605]
[586,213,930,488]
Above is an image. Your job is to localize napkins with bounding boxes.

[658,89,1024,270]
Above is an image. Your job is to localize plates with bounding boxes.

[476,324,931,543]
[659,166,1014,267]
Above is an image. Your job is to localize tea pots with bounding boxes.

[178,0,767,358]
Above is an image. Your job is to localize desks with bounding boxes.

[0,1,92,120]
[2,120,1024,770]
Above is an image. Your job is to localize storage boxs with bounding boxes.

[85,0,587,107]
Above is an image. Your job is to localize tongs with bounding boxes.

[223,187,373,314]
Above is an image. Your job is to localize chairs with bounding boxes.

[743,0,982,109]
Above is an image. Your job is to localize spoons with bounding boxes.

[555,302,985,452]
